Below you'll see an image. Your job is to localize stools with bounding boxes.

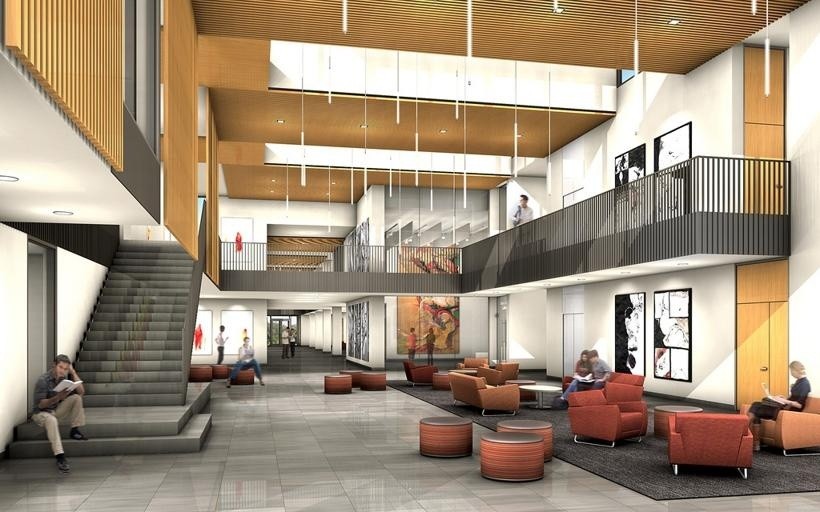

[190,364,254,385]
[432,373,450,390]
[654,406,704,439]
[505,380,536,401]
[420,416,552,482]
[323,370,386,395]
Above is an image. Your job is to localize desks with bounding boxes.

[519,385,562,409]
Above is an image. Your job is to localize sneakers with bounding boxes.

[57,459,71,475]
[70,431,88,440]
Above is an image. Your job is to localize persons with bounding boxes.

[236,232,243,251]
[420,328,435,366]
[747,361,810,452]
[194,324,202,351]
[226,337,265,388]
[589,350,612,390]
[30,355,88,471]
[557,350,594,403]
[399,328,417,361]
[288,328,296,358]
[281,327,290,359]
[512,194,533,225]
[215,325,229,364]
[615,138,679,188]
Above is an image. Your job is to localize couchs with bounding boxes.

[448,372,519,416]
[563,372,645,402]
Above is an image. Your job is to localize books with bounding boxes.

[767,395,786,405]
[52,379,84,392]
[573,373,595,383]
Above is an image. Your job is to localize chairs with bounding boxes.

[567,390,648,448]
[456,357,489,369]
[403,358,438,387]
[740,397,820,457]
[668,411,753,479]
[477,362,519,386]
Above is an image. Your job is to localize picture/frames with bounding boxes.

[192,309,213,356]
[615,292,646,377]
[654,288,692,381]
[221,216,254,264]
[220,310,255,355]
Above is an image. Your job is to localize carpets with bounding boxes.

[386,379,820,501]
